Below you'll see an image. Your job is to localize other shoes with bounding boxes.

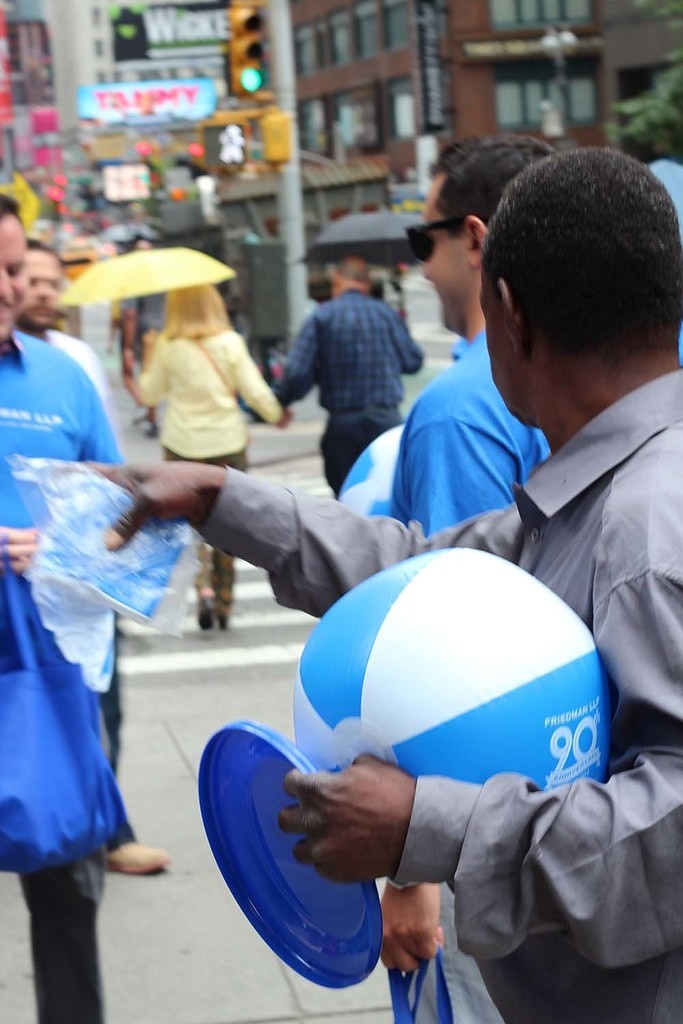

[199,600,214,630]
[104,841,172,875]
[132,406,150,423]
[219,616,227,630]
[144,423,154,434]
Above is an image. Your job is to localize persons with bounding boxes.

[373,133,554,1024]
[92,144,683,1024]
[105,238,169,439]
[125,284,294,632]
[0,194,170,1024]
[252,228,421,504]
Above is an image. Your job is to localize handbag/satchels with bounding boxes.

[0,545,138,873]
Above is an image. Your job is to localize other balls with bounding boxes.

[336,421,405,521]
[292,546,613,791]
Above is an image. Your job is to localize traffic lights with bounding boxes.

[196,116,253,170]
[222,6,265,97]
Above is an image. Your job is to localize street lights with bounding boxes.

[537,26,582,151]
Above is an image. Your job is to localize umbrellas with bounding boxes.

[52,248,235,306]
[301,212,429,267]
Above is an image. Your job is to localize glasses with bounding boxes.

[408,216,489,261]
